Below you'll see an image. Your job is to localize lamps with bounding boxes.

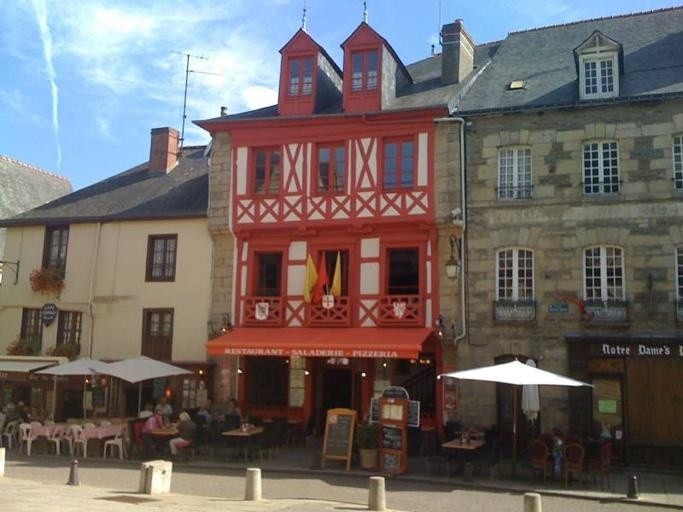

[444,233,462,281]
[305,368,312,377]
[360,368,369,380]
[236,368,245,374]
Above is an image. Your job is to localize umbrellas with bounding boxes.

[521,359,540,422]
[90,354,195,418]
[440,358,595,479]
[35,357,108,417]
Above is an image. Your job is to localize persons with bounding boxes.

[136,394,242,463]
[1,401,34,452]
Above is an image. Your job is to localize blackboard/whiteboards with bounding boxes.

[322,408,357,459]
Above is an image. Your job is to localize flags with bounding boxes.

[313,251,329,304]
[330,250,341,297]
[304,253,319,302]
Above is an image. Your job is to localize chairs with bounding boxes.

[0,416,145,461]
[527,427,625,493]
[425,427,458,478]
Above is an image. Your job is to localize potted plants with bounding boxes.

[355,423,381,470]
